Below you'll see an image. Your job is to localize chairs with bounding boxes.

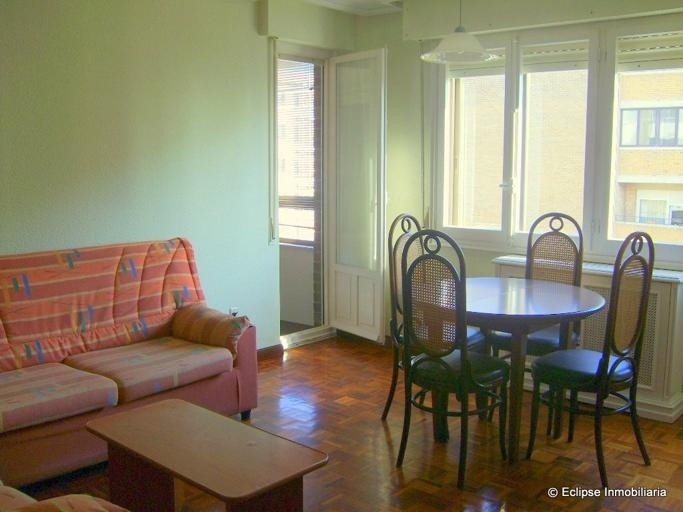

[521,228,658,491]
[0,473,135,512]
[482,211,592,435]
[393,227,513,491]
[379,210,495,423]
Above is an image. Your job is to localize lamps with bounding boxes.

[419,0,490,65]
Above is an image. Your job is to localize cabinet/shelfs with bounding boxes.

[486,252,682,426]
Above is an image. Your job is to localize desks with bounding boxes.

[396,277,608,468]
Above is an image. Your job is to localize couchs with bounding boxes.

[2,226,268,488]
